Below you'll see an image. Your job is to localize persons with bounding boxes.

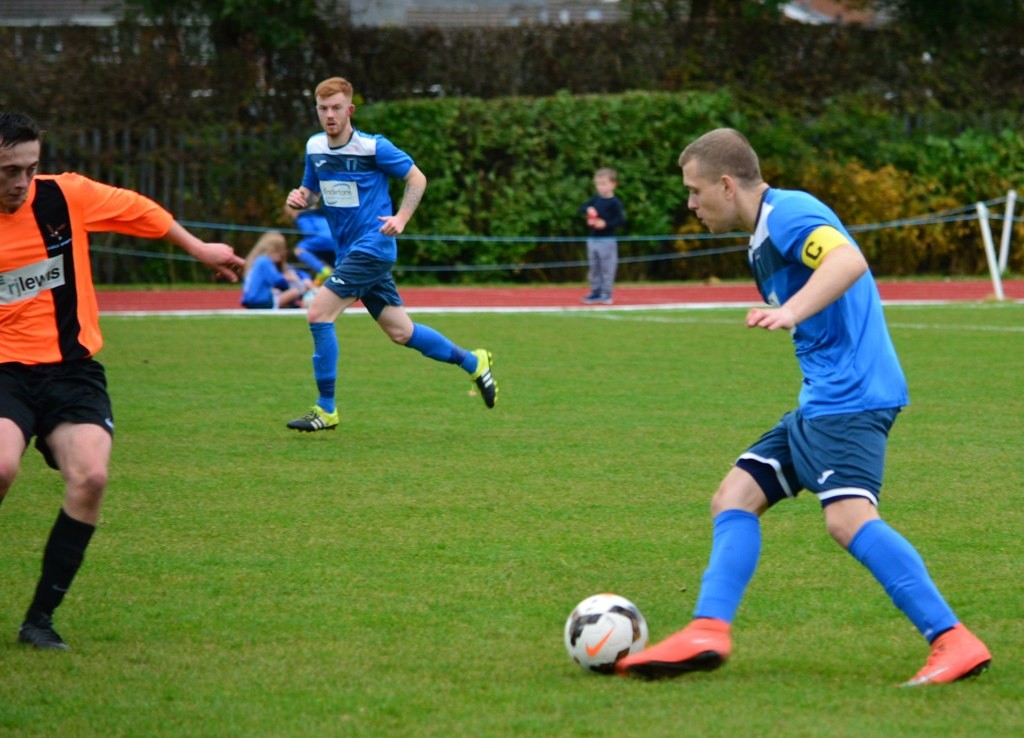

[0,109,246,651]
[239,202,336,309]
[615,127,994,686]
[285,77,498,432]
[578,168,621,304]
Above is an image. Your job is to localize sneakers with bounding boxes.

[902,624,992,690]
[16,620,69,652]
[614,615,731,680]
[287,397,339,431]
[469,348,496,407]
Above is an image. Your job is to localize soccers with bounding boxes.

[564,593,650,674]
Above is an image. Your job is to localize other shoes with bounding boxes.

[584,288,612,305]
